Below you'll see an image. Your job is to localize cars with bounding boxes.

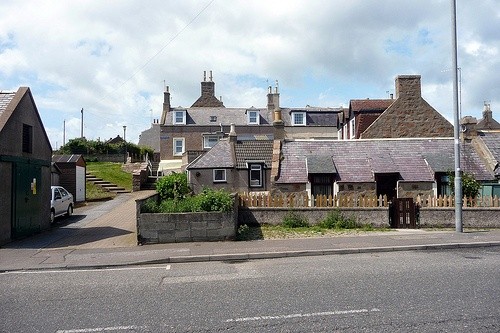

[50,186,74,222]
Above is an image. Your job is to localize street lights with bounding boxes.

[123,126,126,164]
